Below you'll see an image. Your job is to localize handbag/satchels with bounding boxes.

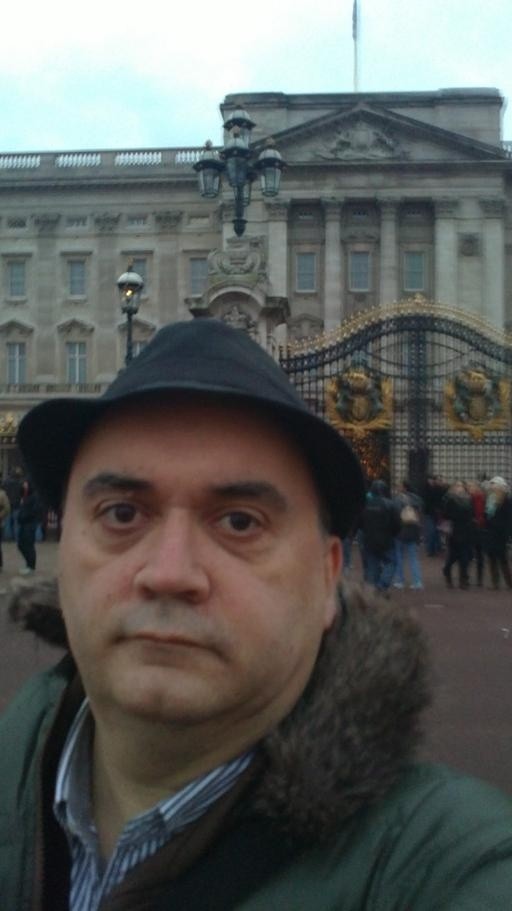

[399,504,419,526]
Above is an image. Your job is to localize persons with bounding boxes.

[0,464,64,577]
[0,318,511,910]
[339,473,512,599]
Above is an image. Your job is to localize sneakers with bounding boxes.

[393,581,422,590]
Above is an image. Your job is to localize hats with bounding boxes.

[16,319,367,544]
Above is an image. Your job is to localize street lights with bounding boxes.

[113,265,146,370]
